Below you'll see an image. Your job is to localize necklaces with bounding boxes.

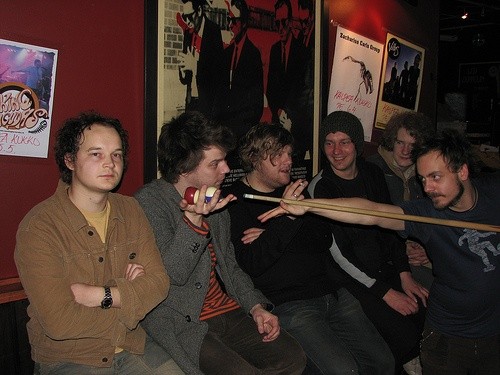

[468,183,476,211]
[244,176,250,187]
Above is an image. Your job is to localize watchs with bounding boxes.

[101,286,113,309]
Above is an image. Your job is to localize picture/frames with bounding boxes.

[143,0,327,193]
[373,30,431,133]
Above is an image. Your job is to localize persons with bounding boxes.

[11,60,42,94]
[177,0,314,170]
[365,112,445,291]
[221,121,395,375]
[307,111,429,375]
[257,139,500,375]
[382,54,421,110]
[14,112,186,375]
[131,111,307,375]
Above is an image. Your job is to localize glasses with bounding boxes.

[226,16,241,25]
[274,17,290,29]
[182,9,198,22]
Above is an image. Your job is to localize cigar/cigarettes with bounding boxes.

[300,183,306,186]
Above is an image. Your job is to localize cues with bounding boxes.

[243,193,500,233]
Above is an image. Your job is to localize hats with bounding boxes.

[319,111,365,152]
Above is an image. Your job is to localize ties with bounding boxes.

[185,32,199,104]
[230,43,238,83]
[282,46,287,76]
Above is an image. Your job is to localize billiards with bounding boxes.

[182,186,199,205]
[204,186,218,204]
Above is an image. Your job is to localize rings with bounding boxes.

[293,194,299,198]
